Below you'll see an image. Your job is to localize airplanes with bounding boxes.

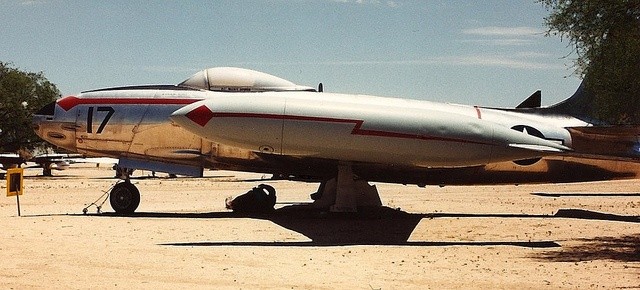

[27,67,637,216]
